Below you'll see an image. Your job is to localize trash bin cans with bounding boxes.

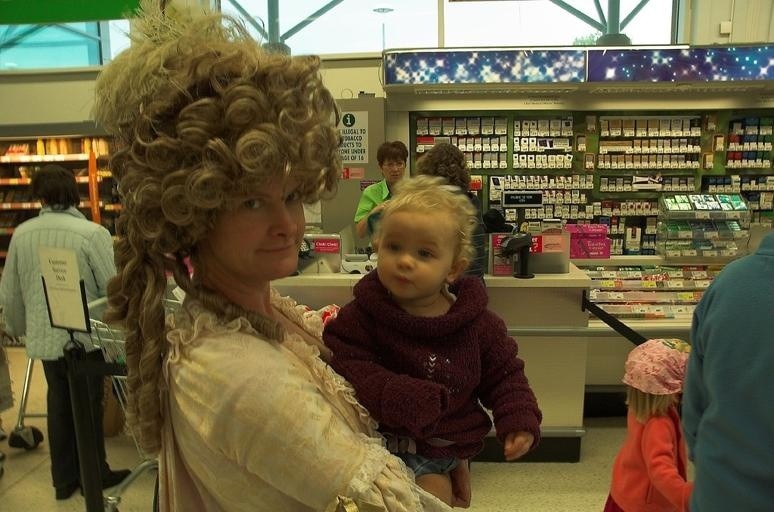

[101,376,126,437]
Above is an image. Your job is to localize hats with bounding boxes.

[621,338,691,395]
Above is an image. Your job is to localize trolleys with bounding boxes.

[73,295,164,512]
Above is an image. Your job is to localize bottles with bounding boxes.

[35,138,109,155]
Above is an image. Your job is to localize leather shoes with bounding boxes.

[56,475,78,499]
[79,461,131,496]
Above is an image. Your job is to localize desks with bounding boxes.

[383,262,591,463]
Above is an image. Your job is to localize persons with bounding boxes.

[0,164,132,500]
[603,337,694,512]
[680,227,774,512]
[92,10,454,512]
[353,140,409,242]
[319,176,541,508]
[414,142,486,278]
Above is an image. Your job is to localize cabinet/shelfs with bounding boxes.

[381,46,774,391]
[0,122,119,269]
